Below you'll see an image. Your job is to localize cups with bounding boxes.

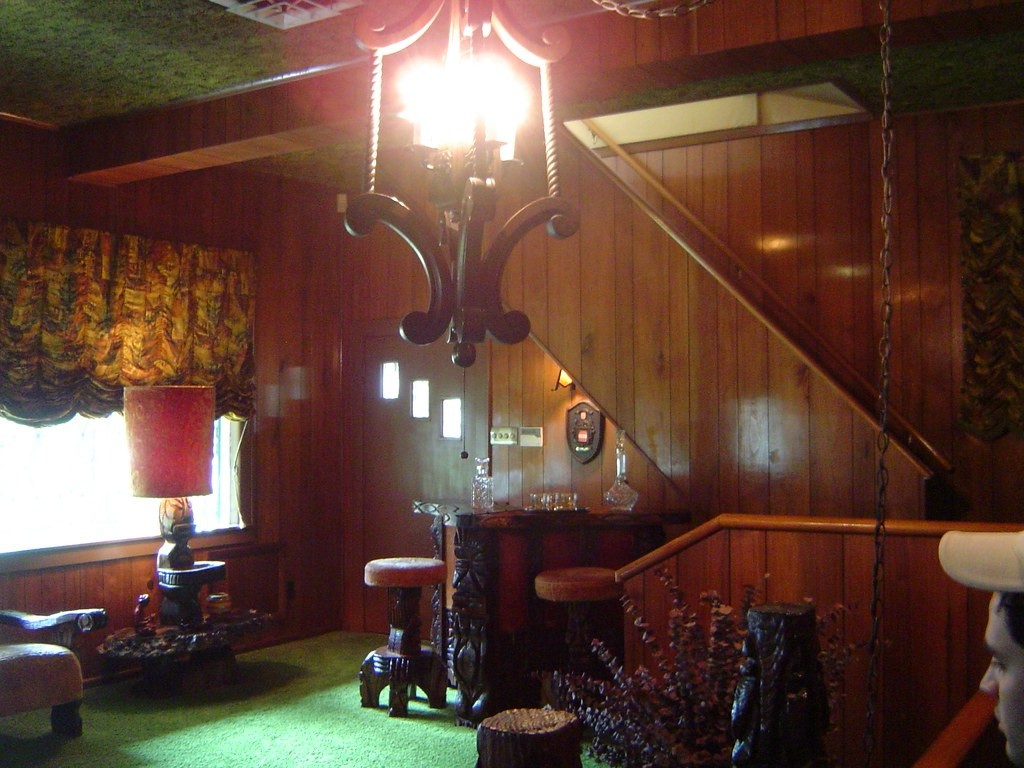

[529,491,578,510]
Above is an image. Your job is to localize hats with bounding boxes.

[938,530,1024,592]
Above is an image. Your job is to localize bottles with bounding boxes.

[470,458,495,510]
[604,430,638,512]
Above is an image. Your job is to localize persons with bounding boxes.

[938,529,1024,766]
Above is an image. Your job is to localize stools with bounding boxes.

[0,608,107,737]
[534,567,624,711]
[359,555,452,718]
[476,707,584,767]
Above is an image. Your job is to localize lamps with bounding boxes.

[122,383,213,569]
[343,0,577,367]
